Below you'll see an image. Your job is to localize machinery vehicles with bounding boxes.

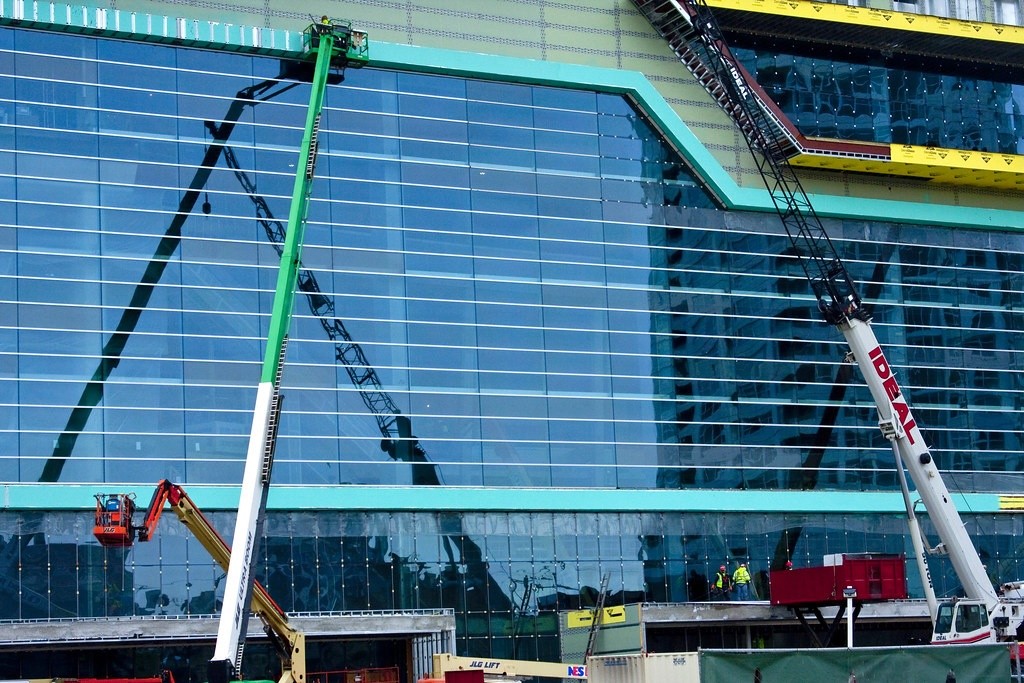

[96,477,588,683]
[683,0,1023,648]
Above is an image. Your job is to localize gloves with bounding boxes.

[711,584,714,590]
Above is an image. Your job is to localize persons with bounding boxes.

[733,564,751,600]
[319,16,333,24]
[712,565,732,601]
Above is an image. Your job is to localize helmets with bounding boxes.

[741,564,746,567]
[720,565,725,570]
